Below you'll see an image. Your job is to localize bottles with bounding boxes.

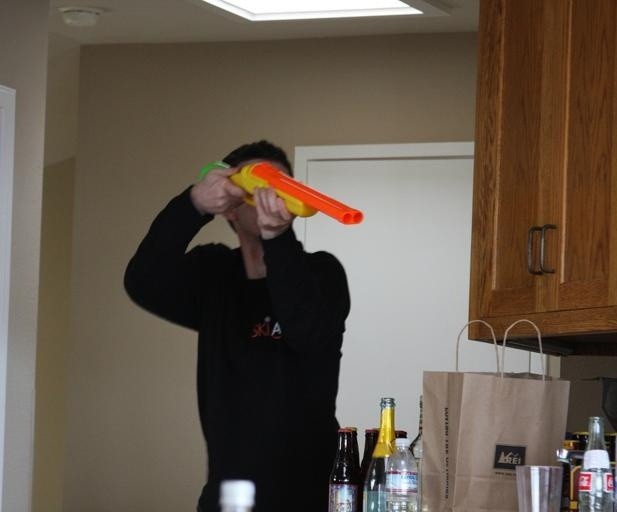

[556,416,615,512]
[219,477,255,512]
[331,395,420,512]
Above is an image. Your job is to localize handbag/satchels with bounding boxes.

[420,318,570,511]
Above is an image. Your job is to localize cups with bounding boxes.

[515,465,562,511]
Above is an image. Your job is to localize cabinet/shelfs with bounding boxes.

[467,0,617,358]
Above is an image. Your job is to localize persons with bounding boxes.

[122,138,355,512]
